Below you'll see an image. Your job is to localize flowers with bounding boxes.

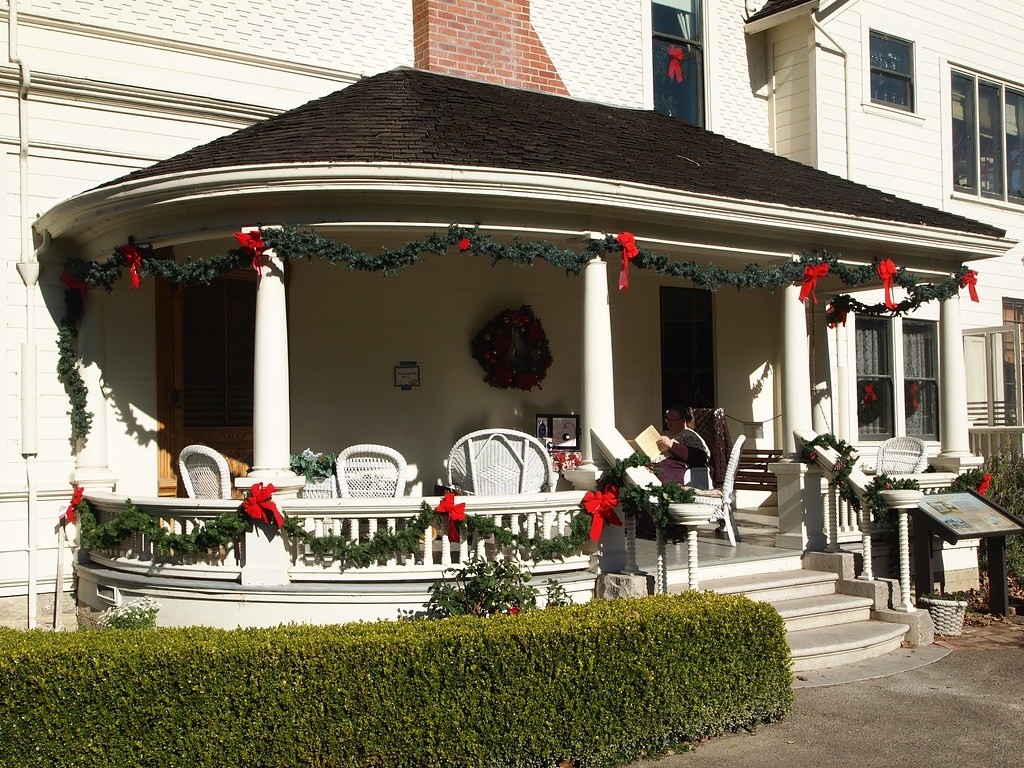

[554,452,583,475]
[291,449,337,478]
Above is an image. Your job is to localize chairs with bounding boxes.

[876,436,929,476]
[335,443,405,501]
[673,435,746,547]
[446,428,560,496]
[178,444,232,501]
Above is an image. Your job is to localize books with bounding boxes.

[625,425,670,461]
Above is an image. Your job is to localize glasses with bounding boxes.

[665,415,684,423]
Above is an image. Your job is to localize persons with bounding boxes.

[640,405,711,487]
